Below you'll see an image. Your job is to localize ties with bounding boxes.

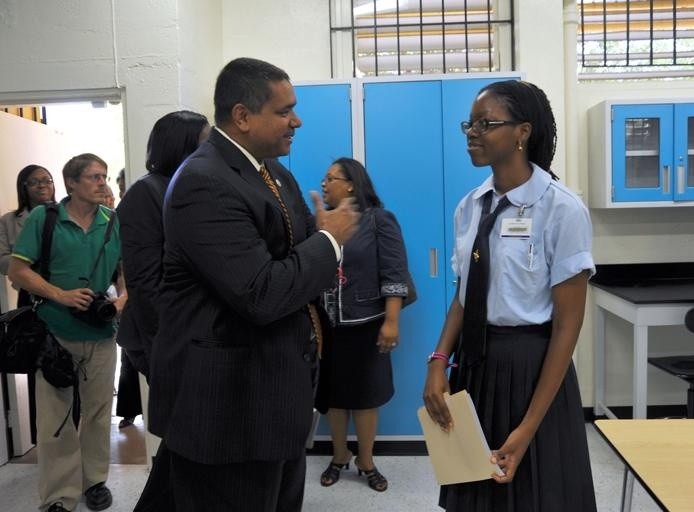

[463,190,512,371]
[259,168,325,362]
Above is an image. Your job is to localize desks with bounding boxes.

[585,262,694,418]
[593,418,693,512]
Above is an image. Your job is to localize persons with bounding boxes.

[422,80,594,508]
[118,111,208,389]
[118,168,143,429]
[314,157,410,492]
[9,153,129,512]
[158,57,361,510]
[0,164,55,273]
[104,186,115,210]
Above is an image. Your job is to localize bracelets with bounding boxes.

[425,353,459,369]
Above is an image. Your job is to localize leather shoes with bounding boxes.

[86,484,112,510]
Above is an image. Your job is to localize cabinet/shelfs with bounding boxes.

[587,98,694,210]
[274,80,356,444]
[358,70,519,440]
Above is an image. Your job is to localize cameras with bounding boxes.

[69,290,117,325]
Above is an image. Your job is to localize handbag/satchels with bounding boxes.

[371,206,417,308]
[0,201,59,373]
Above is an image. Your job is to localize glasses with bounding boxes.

[460,117,523,134]
[23,177,53,186]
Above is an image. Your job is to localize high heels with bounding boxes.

[119,411,143,428]
[319,461,350,487]
[353,456,388,492]
